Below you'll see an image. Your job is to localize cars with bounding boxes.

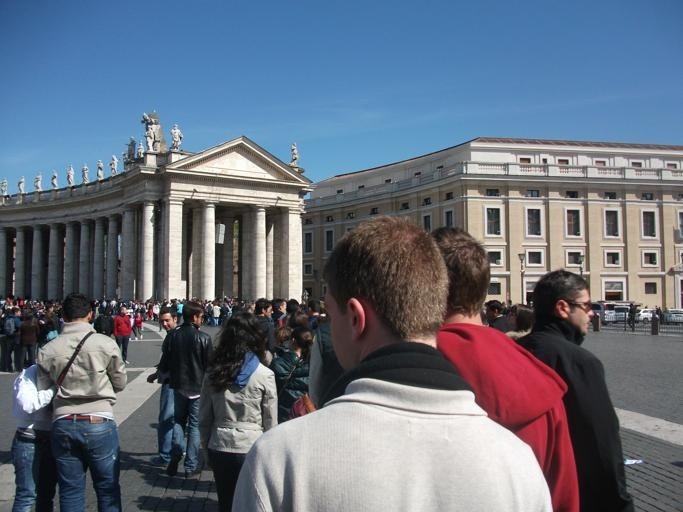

[589,299,683,326]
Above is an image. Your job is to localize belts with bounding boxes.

[60,413,110,422]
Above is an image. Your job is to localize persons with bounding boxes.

[1,294,343,511]
[625,303,664,331]
[232,216,635,510]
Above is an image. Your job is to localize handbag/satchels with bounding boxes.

[289,392,316,419]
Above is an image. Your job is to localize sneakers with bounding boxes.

[149,454,206,478]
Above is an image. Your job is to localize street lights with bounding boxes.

[517,249,527,306]
[578,250,586,279]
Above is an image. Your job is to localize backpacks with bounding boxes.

[4,316,17,336]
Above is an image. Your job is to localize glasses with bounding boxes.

[565,301,592,313]
